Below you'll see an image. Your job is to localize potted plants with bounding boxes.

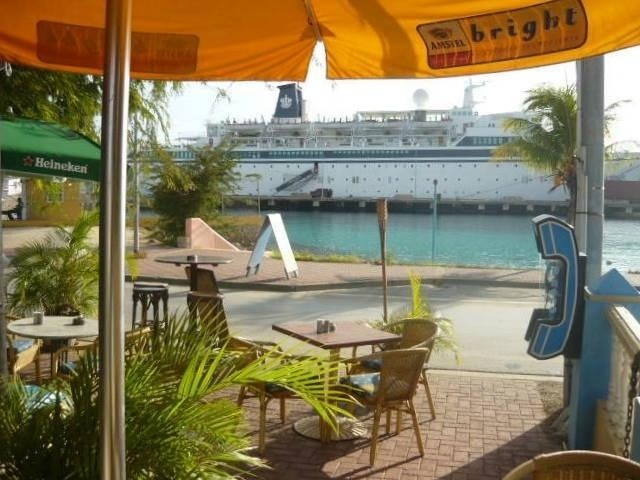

[3,208,139,352]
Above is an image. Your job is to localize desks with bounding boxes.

[271,319,402,442]
[154,254,233,329]
[7,315,99,353]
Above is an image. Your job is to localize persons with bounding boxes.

[0,197,24,221]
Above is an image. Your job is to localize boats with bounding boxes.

[126,79,640,211]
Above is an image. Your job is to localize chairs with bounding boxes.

[50,327,150,378]
[327,348,428,466]
[352,318,438,431]
[500,449,640,480]
[230,336,324,456]
[6,330,41,388]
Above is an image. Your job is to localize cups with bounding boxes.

[32,311,44,326]
[316,319,329,334]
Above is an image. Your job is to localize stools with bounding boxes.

[132,285,168,343]
[188,290,224,339]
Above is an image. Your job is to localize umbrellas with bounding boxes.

[0,0,640,477]
